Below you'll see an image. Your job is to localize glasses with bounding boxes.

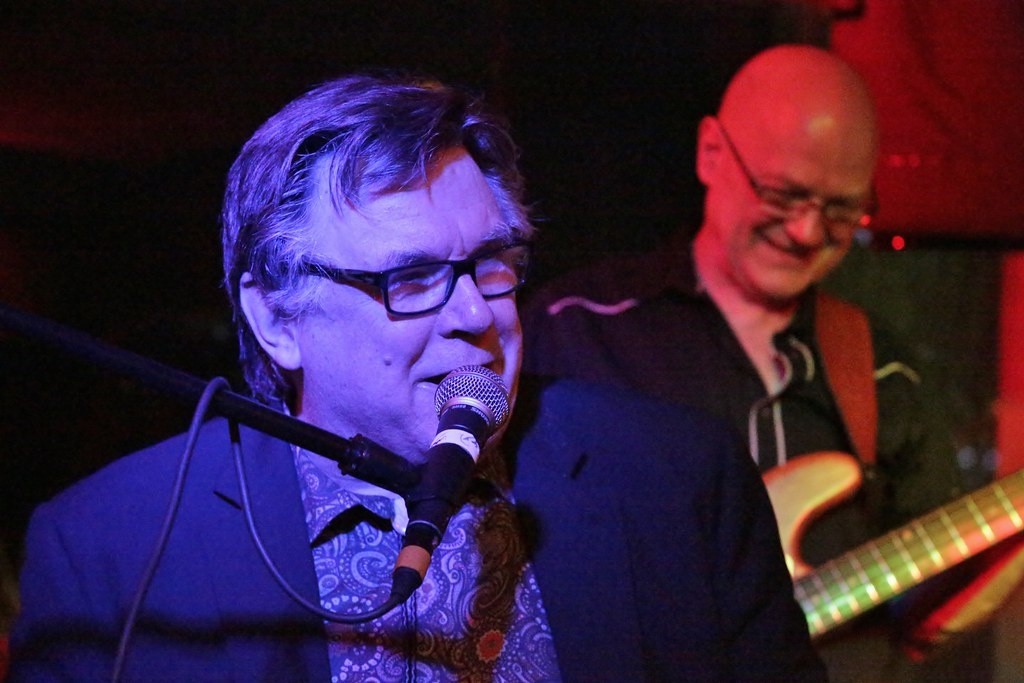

[230,240,534,318]
[723,131,881,242]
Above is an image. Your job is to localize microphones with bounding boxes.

[392,365,511,603]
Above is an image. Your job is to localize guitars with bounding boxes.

[761,451,1024,639]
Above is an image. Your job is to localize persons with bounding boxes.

[599,41,996,683]
[13,72,833,682]
